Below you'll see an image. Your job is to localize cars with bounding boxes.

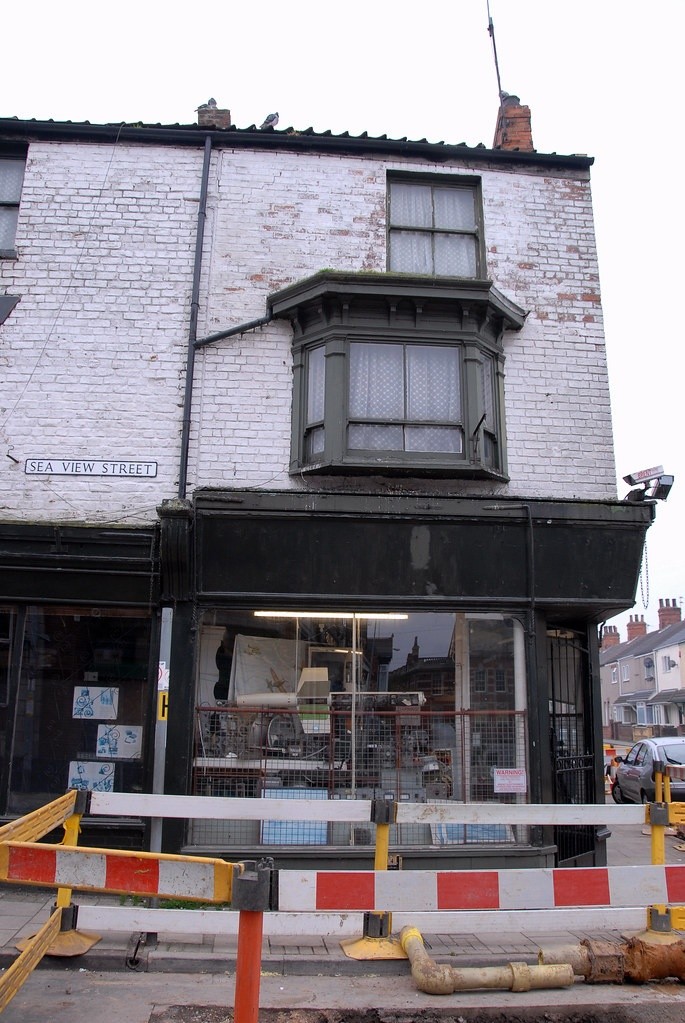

[612,736,685,804]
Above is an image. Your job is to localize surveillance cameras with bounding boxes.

[651,475,675,500]
[623,466,665,486]
